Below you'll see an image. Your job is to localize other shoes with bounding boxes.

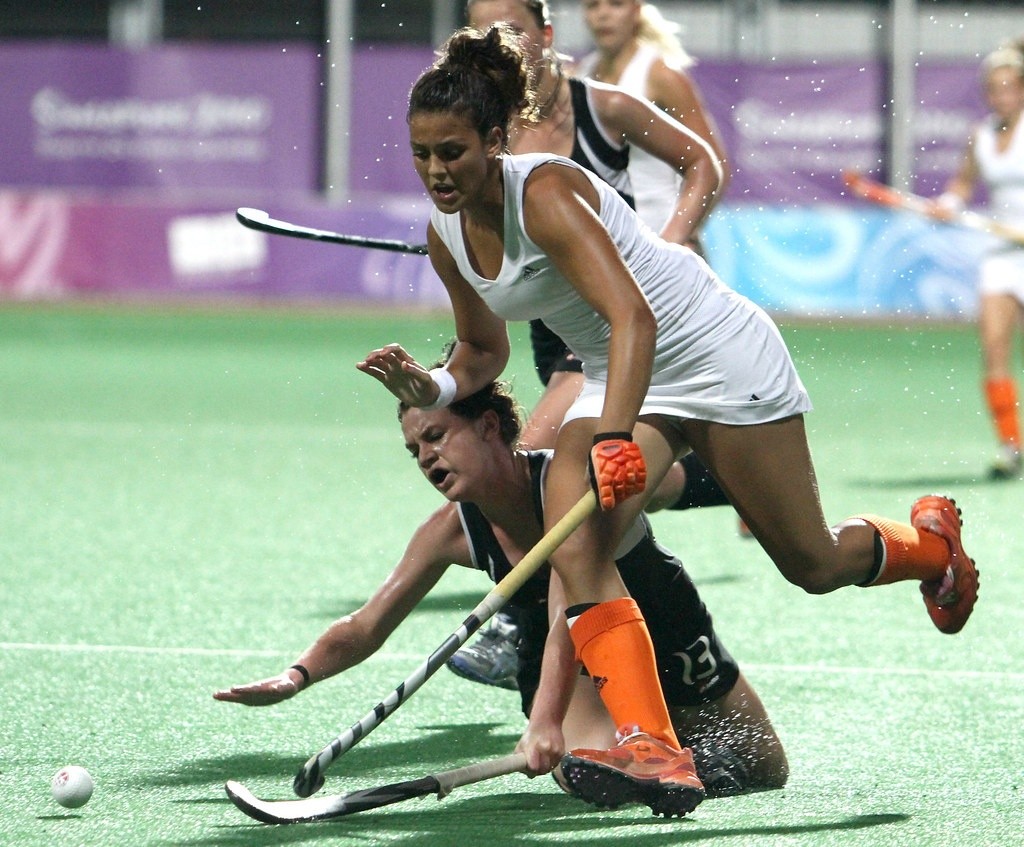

[991,444,1019,474]
[741,519,753,537]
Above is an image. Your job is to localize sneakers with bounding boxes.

[910,494,980,634]
[551,725,706,818]
[446,612,520,691]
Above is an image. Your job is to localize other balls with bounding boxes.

[51,766,94,809]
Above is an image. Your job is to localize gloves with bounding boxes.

[587,431,648,511]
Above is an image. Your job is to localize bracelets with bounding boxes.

[421,368,457,411]
[937,192,967,210]
[290,665,310,691]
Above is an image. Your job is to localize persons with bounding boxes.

[930,40,1024,480]
[357,21,980,818]
[446,0,731,693]
[214,340,788,796]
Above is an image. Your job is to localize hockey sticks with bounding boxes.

[224,751,536,826]
[290,481,605,801]
[235,206,429,257]
[841,169,1024,248]
